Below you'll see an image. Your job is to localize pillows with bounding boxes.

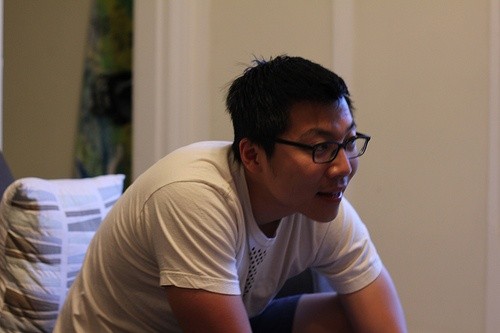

[0,173,125,333]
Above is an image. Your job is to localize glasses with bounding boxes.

[276,130,372,163]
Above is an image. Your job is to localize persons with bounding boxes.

[54,51,406,332]
